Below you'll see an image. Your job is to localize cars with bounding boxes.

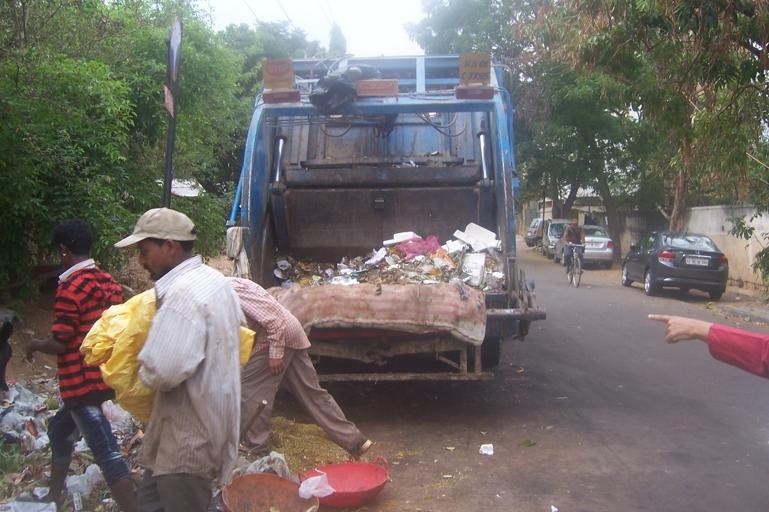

[622,230,730,300]
[554,224,613,267]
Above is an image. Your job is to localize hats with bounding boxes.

[113,207,196,248]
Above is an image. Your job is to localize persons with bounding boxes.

[24,219,139,511]
[649,312,769,381]
[562,218,586,274]
[221,276,373,461]
[112,206,244,512]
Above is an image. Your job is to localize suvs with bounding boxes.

[526,216,543,246]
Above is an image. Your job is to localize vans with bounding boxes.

[542,219,571,259]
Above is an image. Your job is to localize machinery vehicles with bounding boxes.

[222,51,550,381]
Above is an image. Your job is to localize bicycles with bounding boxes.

[565,242,587,288]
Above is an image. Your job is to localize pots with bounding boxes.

[300,455,390,509]
[221,472,321,512]
